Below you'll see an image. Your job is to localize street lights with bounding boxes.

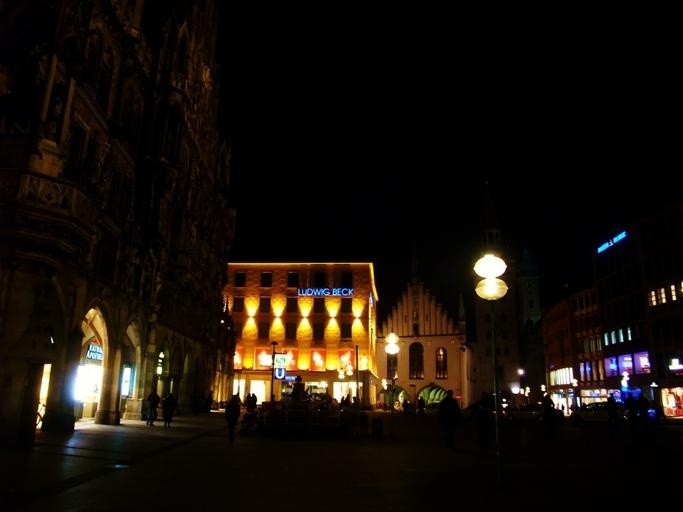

[472,253,509,446]
[269,341,287,404]
[383,332,400,411]
[539,384,546,403]
[570,378,579,408]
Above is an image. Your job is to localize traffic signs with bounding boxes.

[337,367,344,396]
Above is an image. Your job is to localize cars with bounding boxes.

[512,405,544,426]
[568,403,623,427]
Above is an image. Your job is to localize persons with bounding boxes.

[403,390,650,473]
[146,375,305,448]
